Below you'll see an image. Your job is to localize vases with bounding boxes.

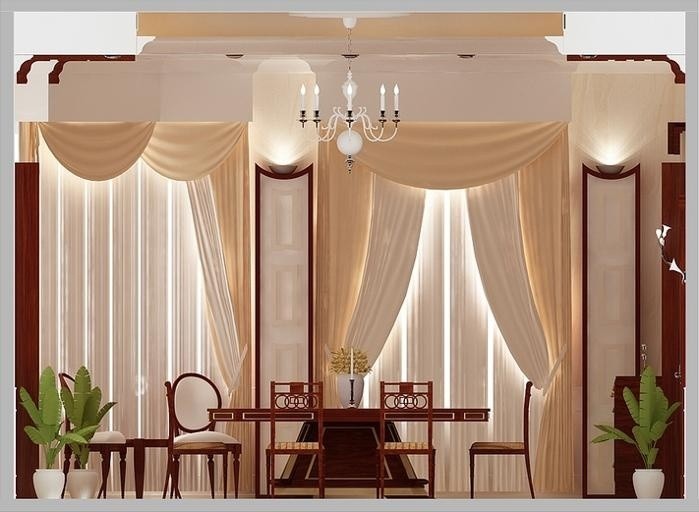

[326,372,371,408]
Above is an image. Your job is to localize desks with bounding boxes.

[203,405,499,498]
[124,436,175,499]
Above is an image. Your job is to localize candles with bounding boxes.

[349,348,355,380]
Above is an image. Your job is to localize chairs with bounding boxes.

[58,373,128,499]
[265,380,328,498]
[467,381,537,499]
[376,380,438,498]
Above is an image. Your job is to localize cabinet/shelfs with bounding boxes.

[612,373,682,498]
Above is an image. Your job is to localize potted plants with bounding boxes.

[59,366,118,498]
[19,366,71,499]
[591,367,682,498]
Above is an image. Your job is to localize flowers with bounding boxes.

[328,344,372,374]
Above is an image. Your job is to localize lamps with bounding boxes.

[593,164,623,176]
[289,9,414,172]
[267,163,298,175]
[654,223,684,286]
